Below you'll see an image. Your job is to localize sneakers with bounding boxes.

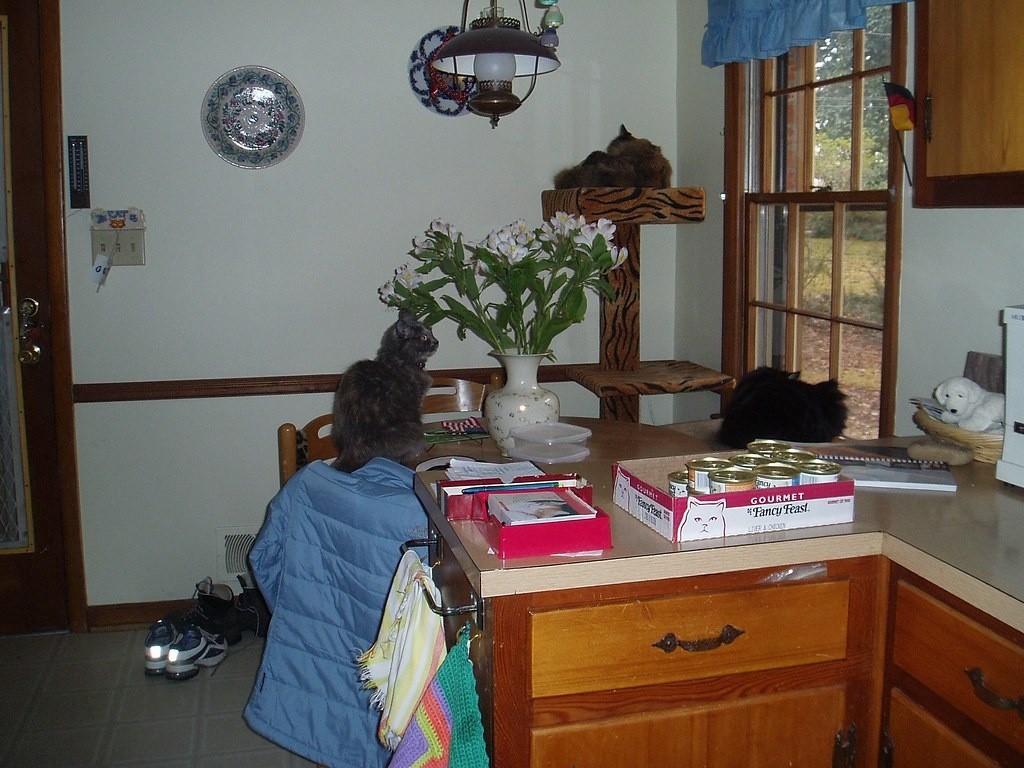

[165,624,228,680]
[144,620,179,676]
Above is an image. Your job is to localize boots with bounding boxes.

[175,576,243,646]
[236,573,272,638]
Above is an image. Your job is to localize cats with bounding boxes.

[553,123,673,188]
[714,365,850,450]
[329,309,439,474]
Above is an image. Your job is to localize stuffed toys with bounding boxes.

[937,377,1004,433]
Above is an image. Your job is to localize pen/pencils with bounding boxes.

[462,481,564,494]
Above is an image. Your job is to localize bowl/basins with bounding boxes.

[507,446,591,475]
[509,420,592,458]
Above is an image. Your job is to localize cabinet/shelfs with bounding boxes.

[913,0,1024,210]
[428,428,1024,768]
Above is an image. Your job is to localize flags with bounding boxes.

[442,416,485,435]
[884,82,915,131]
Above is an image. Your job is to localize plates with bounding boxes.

[200,65,304,170]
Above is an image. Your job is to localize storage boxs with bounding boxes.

[611,450,855,545]
[436,474,613,560]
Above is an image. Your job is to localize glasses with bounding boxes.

[423,429,483,452]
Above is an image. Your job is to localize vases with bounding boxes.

[486,348,561,458]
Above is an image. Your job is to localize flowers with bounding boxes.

[377,210,629,362]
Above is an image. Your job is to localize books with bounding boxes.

[818,455,957,491]
[487,492,597,527]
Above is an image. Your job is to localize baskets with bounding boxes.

[913,410,1004,465]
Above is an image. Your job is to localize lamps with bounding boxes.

[431,0,561,130]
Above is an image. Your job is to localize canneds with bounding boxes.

[668,440,841,498]
